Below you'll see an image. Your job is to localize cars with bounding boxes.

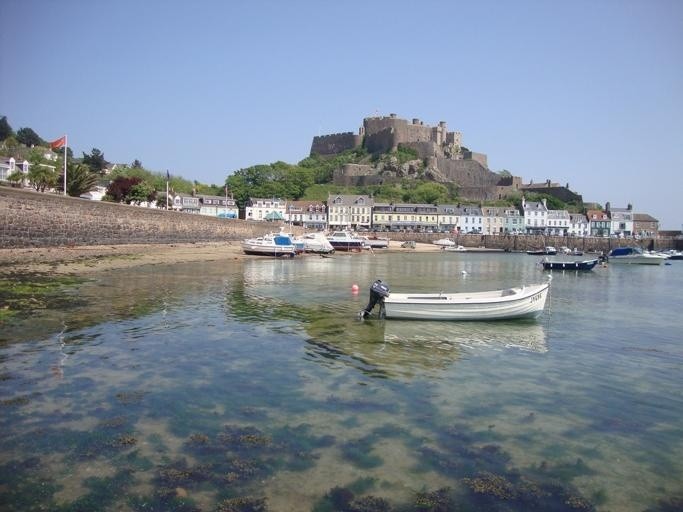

[467,229,484,235]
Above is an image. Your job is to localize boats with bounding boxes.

[432,237,457,246]
[238,230,392,257]
[358,275,554,321]
[521,244,683,272]
[371,317,552,361]
[441,243,468,252]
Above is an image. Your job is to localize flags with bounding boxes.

[49,136,65,149]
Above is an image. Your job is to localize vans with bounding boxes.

[356,227,464,233]
[399,240,416,248]
